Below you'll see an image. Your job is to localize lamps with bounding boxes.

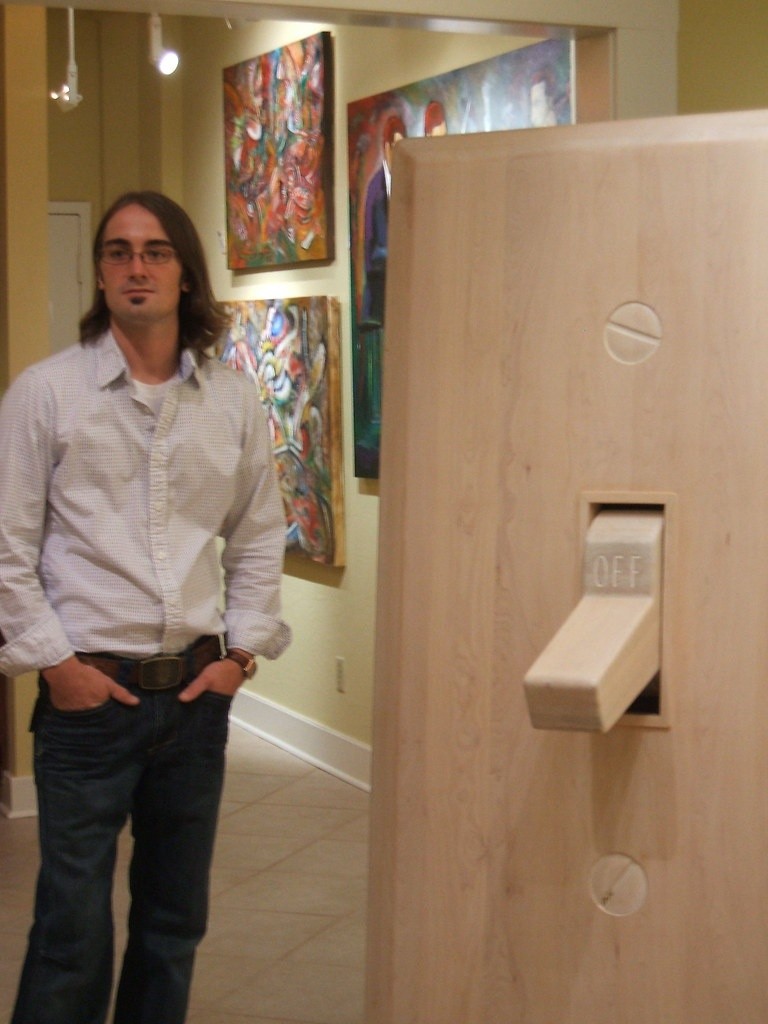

[56,6,83,112]
[140,13,179,75]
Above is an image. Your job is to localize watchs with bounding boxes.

[224,652,256,679]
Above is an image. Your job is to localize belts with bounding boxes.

[76,636,220,691]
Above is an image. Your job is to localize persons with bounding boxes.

[0,190,292,1024]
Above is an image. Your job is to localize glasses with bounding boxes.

[96,246,179,266]
